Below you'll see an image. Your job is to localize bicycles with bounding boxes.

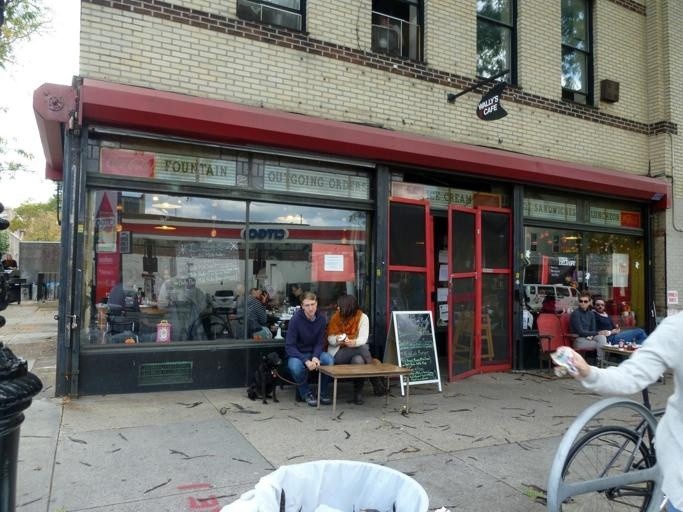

[184,312,233,340]
[547,370,673,512]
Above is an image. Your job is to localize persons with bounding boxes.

[590,294,648,364]
[1,253,17,271]
[108,266,141,334]
[569,290,610,368]
[550,309,683,512]
[379,16,405,48]
[285,292,334,405]
[327,294,389,406]
[244,282,273,340]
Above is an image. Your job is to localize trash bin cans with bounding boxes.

[257,460,429,512]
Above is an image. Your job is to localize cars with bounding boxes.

[211,289,240,313]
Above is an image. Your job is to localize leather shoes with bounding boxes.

[354,390,364,404]
[374,382,384,397]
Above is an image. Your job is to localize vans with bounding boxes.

[523,284,593,315]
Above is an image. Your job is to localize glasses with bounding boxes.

[579,300,588,303]
[595,304,604,306]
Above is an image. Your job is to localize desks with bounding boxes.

[318,364,412,420]
[599,344,667,384]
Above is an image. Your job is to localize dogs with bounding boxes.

[246,352,282,404]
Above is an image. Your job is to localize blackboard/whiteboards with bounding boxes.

[393,311,441,386]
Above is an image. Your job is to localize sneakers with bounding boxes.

[304,391,331,406]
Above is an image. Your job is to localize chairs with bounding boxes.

[101,307,142,344]
[536,313,593,376]
